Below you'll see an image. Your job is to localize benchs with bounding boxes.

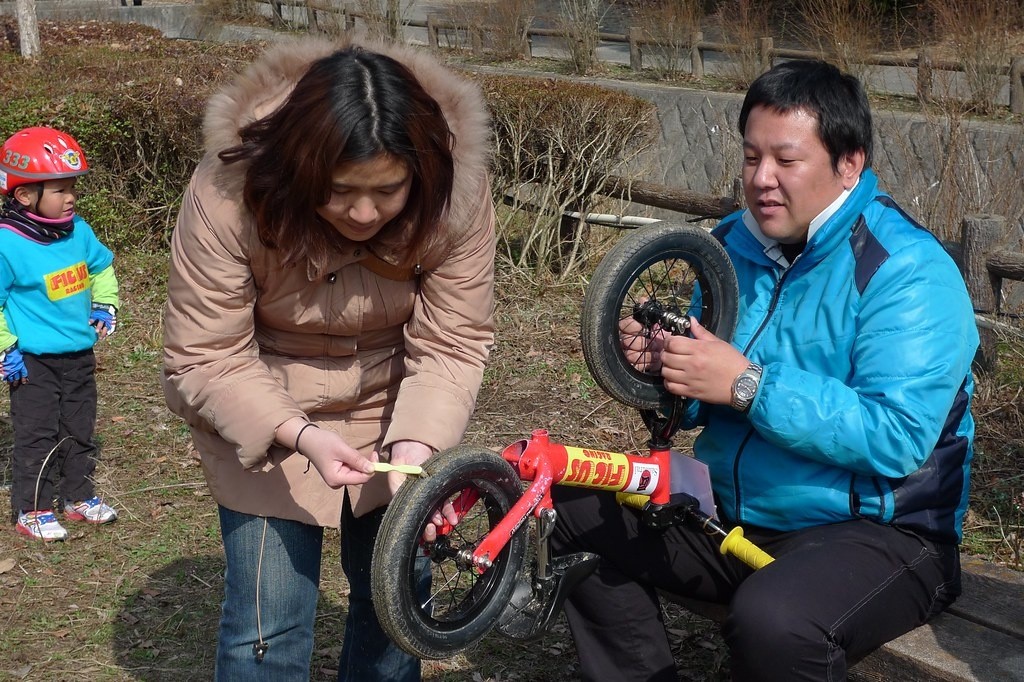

[655,568,1024,682]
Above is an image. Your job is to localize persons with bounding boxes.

[570,59,983,682]
[0,125,123,544]
[159,27,496,682]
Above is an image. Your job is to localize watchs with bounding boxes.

[730,358,763,413]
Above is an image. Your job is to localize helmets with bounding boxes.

[0,126,89,195]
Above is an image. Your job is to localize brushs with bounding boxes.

[371,463,431,479]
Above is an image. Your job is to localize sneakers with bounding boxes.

[14,509,68,541]
[63,496,118,525]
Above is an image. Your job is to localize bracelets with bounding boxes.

[296,423,318,474]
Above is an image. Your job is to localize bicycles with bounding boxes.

[368,220,781,658]
